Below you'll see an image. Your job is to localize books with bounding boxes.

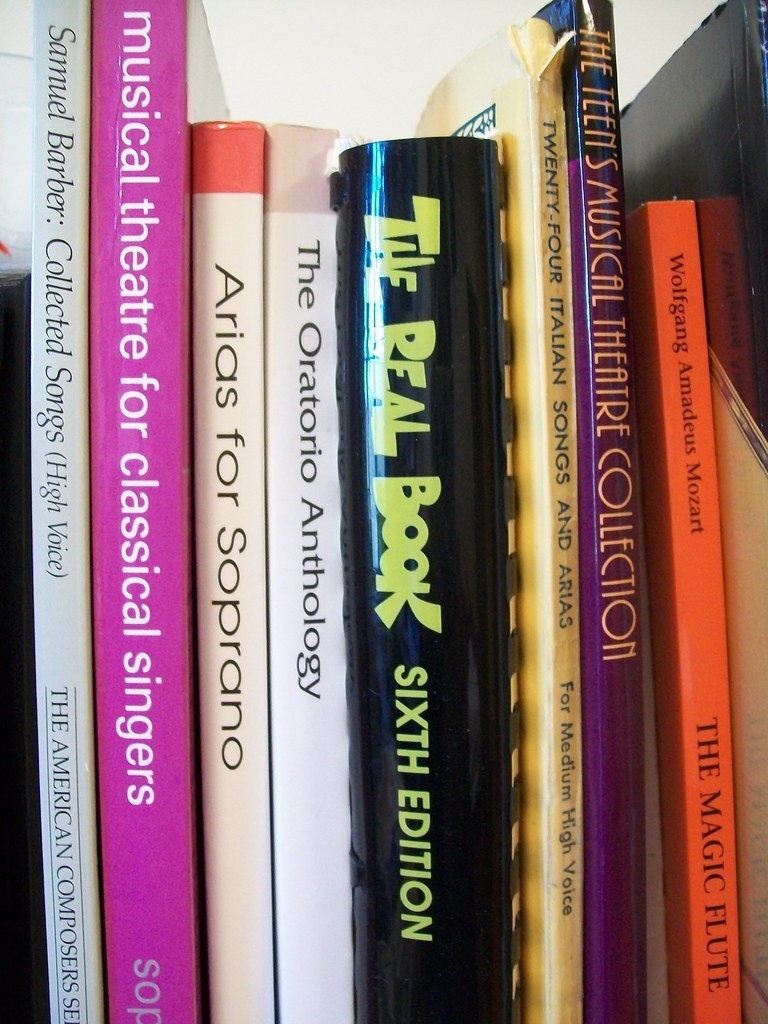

[0,0,768,1024]
